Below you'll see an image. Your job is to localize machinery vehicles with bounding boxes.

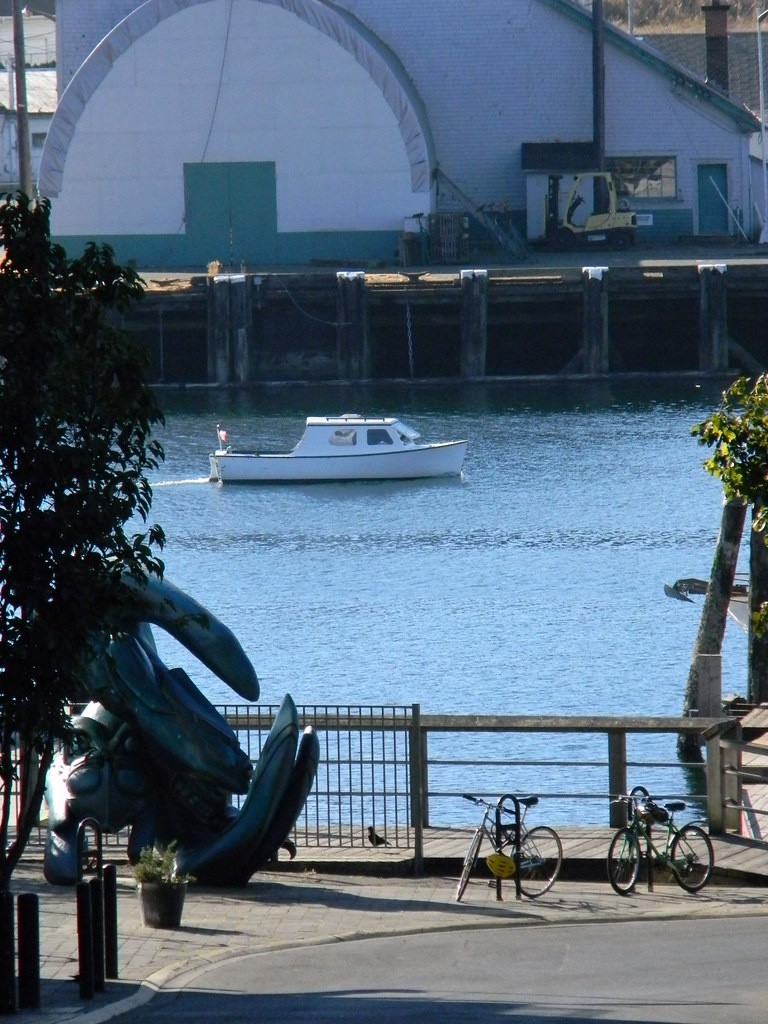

[543,171,639,253]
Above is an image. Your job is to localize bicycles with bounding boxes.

[455,794,563,903]
[607,794,714,895]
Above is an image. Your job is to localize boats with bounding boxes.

[207,412,470,482]
[663,572,751,631]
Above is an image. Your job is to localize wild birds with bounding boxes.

[367,826,392,853]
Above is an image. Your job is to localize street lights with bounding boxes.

[755,11,768,242]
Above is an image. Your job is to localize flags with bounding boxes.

[220,431,226,440]
